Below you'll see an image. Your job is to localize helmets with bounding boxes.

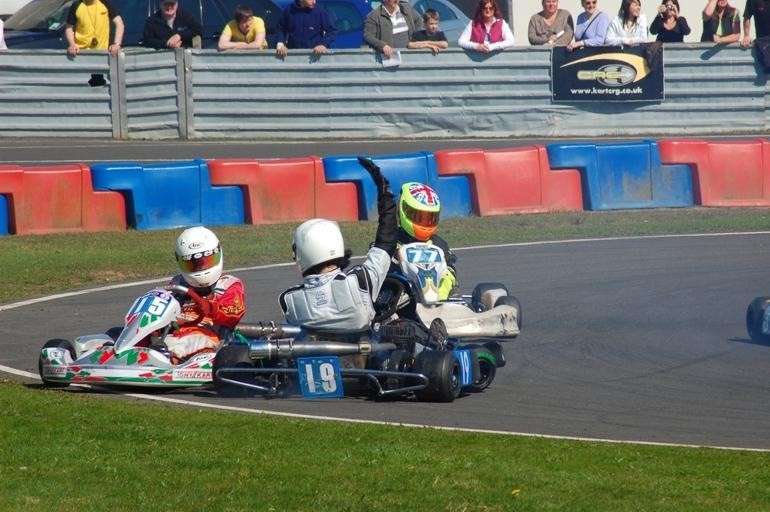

[175,228,222,288]
[398,182,441,241]
[292,218,344,277]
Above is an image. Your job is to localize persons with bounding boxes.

[275,1,336,55]
[649,1,690,43]
[363,0,448,57]
[700,0,741,44]
[143,1,201,49]
[218,7,268,50]
[528,0,573,45]
[741,1,770,47]
[277,156,397,367]
[65,0,124,57]
[606,0,649,44]
[566,0,610,49]
[457,1,516,51]
[369,180,456,305]
[160,225,246,364]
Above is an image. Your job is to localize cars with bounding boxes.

[0,0,381,49]
[370,0,472,48]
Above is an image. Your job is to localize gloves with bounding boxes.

[357,155,394,199]
[193,300,211,317]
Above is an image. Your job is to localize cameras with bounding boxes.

[665,4,672,11]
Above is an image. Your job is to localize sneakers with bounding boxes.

[429,319,448,352]
